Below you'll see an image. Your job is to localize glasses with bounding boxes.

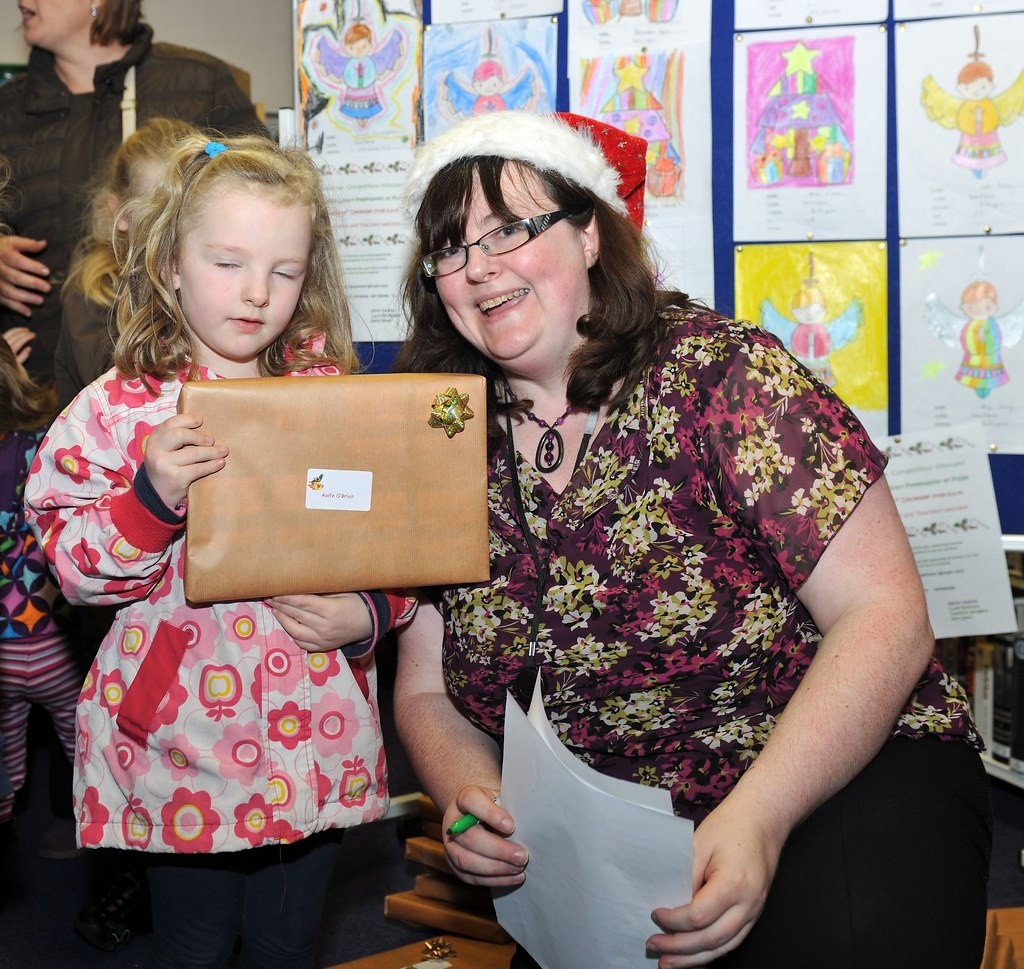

[418,207,571,278]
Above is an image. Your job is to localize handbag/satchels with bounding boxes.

[176,372,491,607]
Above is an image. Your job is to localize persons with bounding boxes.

[0,0,273,422]
[19,114,421,969]
[386,111,987,969]
[0,327,88,902]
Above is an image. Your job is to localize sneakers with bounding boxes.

[74,860,151,952]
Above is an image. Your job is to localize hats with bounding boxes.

[400,110,648,240]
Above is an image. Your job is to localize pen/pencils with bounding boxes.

[446,795,501,836]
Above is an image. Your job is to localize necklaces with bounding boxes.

[503,375,585,473]
